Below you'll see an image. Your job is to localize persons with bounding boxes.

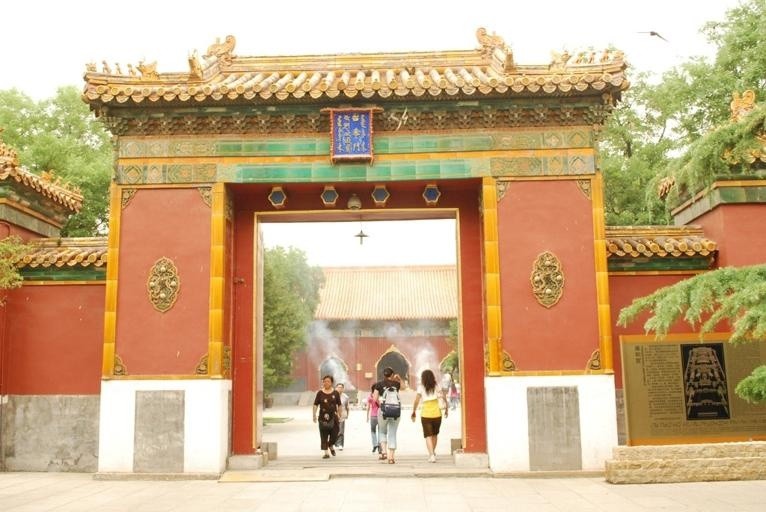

[411,369,449,463]
[312,375,343,459]
[334,383,350,450]
[372,367,406,464]
[367,383,383,454]
[450,383,458,410]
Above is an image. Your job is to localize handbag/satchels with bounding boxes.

[319,416,335,432]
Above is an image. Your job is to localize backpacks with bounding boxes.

[379,382,401,420]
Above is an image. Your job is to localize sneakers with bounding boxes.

[426,454,439,463]
[323,445,344,458]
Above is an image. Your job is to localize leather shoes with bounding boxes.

[371,445,394,464]
